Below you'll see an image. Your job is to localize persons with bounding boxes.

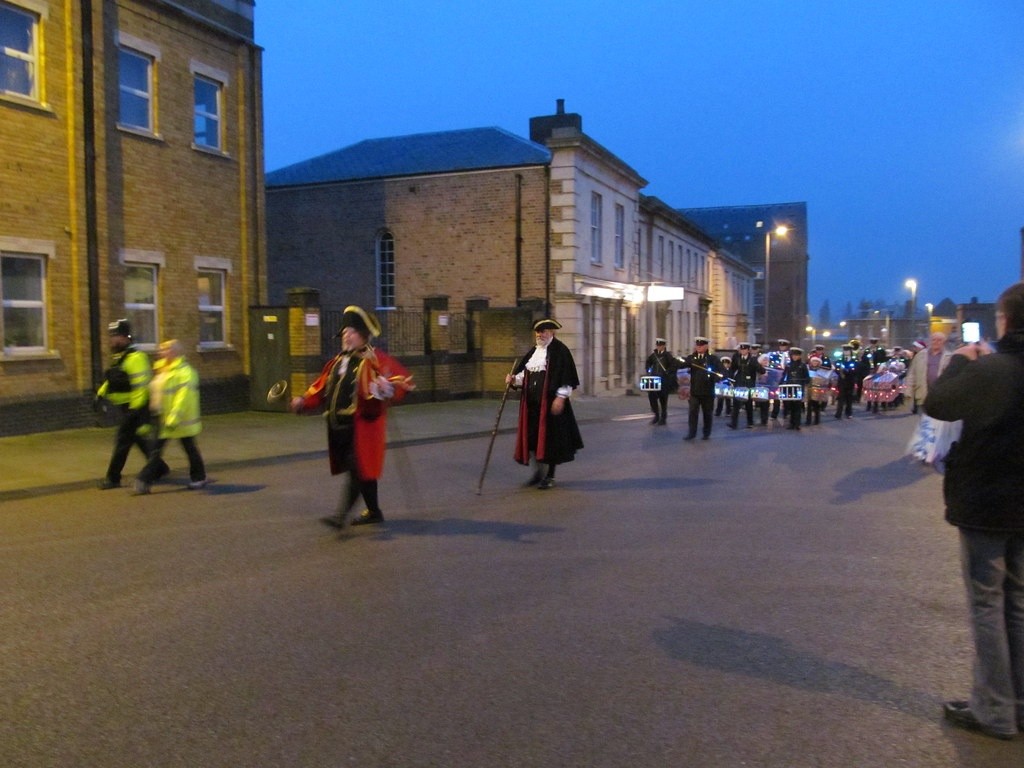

[291,305,417,530]
[642,337,729,440]
[93,318,208,495]
[713,338,839,430]
[506,320,584,491]
[923,282,1024,741]
[903,331,952,466]
[834,338,927,419]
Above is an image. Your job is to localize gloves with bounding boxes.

[93,395,102,412]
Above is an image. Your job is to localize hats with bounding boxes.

[654,335,926,362]
[531,318,562,331]
[342,305,382,337]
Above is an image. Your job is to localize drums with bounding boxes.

[778,384,803,401]
[768,351,790,369]
[639,376,662,392]
[811,387,829,402]
[714,383,750,401]
[750,387,769,402]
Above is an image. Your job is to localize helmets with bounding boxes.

[106,318,129,336]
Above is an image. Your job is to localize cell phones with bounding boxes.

[961,321,982,347]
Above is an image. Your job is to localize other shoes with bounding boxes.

[527,470,556,489]
[353,509,384,525]
[651,399,905,441]
[189,478,207,489]
[155,462,170,476]
[133,477,149,493]
[323,515,343,528]
[98,479,120,490]
[944,700,1018,739]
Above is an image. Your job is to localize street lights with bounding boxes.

[806,326,816,351]
[764,226,788,344]
[905,279,918,348]
